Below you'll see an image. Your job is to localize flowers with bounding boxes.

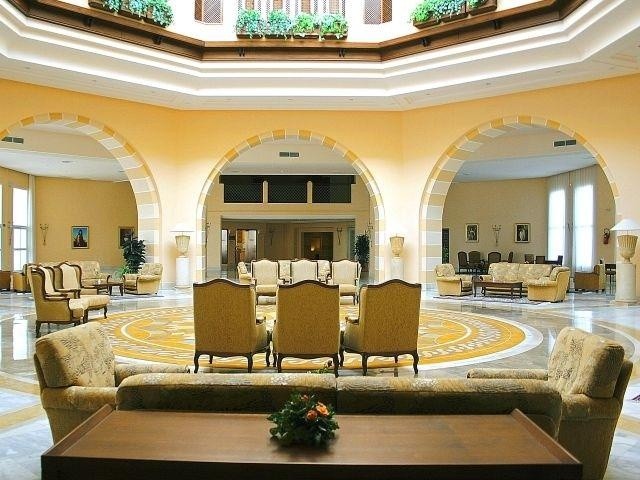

[267,393,338,447]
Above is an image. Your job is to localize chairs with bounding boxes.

[341,279,422,376]
[53,262,110,324]
[290,259,318,284]
[469,250,483,274]
[488,252,501,263]
[317,260,331,279]
[536,256,545,264]
[251,259,280,305]
[192,279,270,374]
[272,278,339,377]
[326,260,359,304]
[458,251,475,274]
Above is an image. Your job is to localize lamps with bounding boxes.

[389,233,405,280]
[170,220,194,290]
[610,220,640,302]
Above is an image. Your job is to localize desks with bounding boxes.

[39,403,582,475]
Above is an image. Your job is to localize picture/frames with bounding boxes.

[466,223,479,243]
[118,226,134,249]
[70,226,89,249]
[514,222,532,243]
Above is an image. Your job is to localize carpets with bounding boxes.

[433,293,543,305]
[87,306,525,371]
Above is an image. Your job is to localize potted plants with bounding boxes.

[122,230,146,278]
[115,263,132,279]
[353,230,369,279]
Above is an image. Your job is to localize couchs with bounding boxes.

[0,269,12,292]
[435,264,472,296]
[479,261,561,296]
[237,261,251,283]
[32,321,633,479]
[13,263,35,294]
[40,261,112,288]
[574,264,606,294]
[123,263,163,296]
[527,267,570,302]
[28,265,84,338]
